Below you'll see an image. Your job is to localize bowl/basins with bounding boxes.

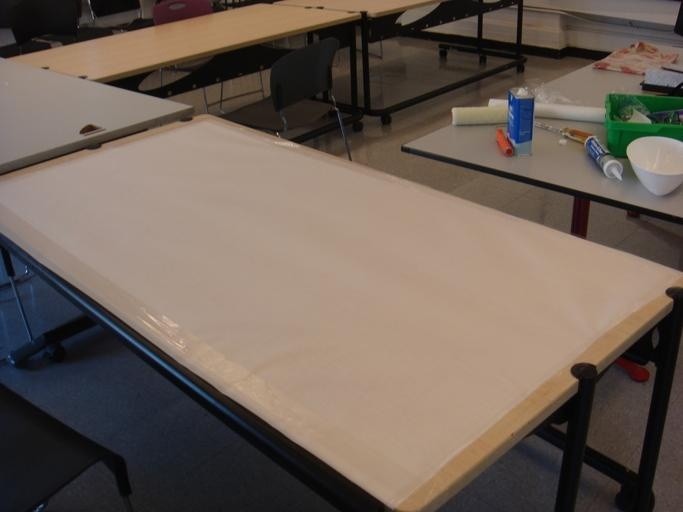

[627,136,683,197]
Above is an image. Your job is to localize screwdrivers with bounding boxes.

[535,122,593,144]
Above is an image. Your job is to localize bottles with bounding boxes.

[584,135,624,182]
